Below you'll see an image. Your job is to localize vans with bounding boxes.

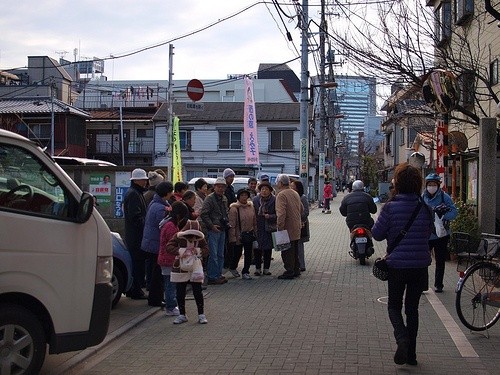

[0,130,115,375]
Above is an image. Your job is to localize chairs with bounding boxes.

[453,232,500,272]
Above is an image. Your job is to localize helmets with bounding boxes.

[409,151,426,169]
[352,180,364,190]
[129,168,149,180]
[425,173,442,185]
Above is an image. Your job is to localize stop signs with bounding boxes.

[187,79,204,101]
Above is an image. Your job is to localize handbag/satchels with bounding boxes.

[372,257,388,281]
[239,229,257,245]
[190,258,204,283]
[272,227,292,251]
[179,250,195,272]
[252,240,259,249]
[434,213,448,238]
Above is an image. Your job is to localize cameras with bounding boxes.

[219,217,230,229]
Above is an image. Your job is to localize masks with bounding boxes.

[427,187,437,194]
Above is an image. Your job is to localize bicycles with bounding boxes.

[453,231,500,338]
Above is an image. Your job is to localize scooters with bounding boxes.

[348,224,375,265]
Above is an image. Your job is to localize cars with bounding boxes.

[189,174,305,196]
[110,231,133,311]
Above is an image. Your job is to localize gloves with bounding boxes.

[432,204,447,214]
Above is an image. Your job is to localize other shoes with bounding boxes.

[131,294,149,299]
[207,276,228,285]
[262,269,272,275]
[198,314,208,324]
[241,273,252,280]
[163,306,180,316]
[393,331,419,365]
[148,301,166,307]
[173,314,188,323]
[254,269,262,275]
[434,284,444,292]
[229,268,241,277]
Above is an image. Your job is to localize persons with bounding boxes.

[422,173,458,292]
[322,178,353,214]
[339,180,377,232]
[371,163,436,366]
[98,175,111,185]
[122,168,310,324]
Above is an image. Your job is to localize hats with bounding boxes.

[223,168,235,178]
[213,177,228,187]
[258,182,273,192]
[148,168,167,186]
[261,174,269,180]
[236,188,251,199]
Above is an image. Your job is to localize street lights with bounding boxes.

[319,115,347,204]
[299,82,339,197]
